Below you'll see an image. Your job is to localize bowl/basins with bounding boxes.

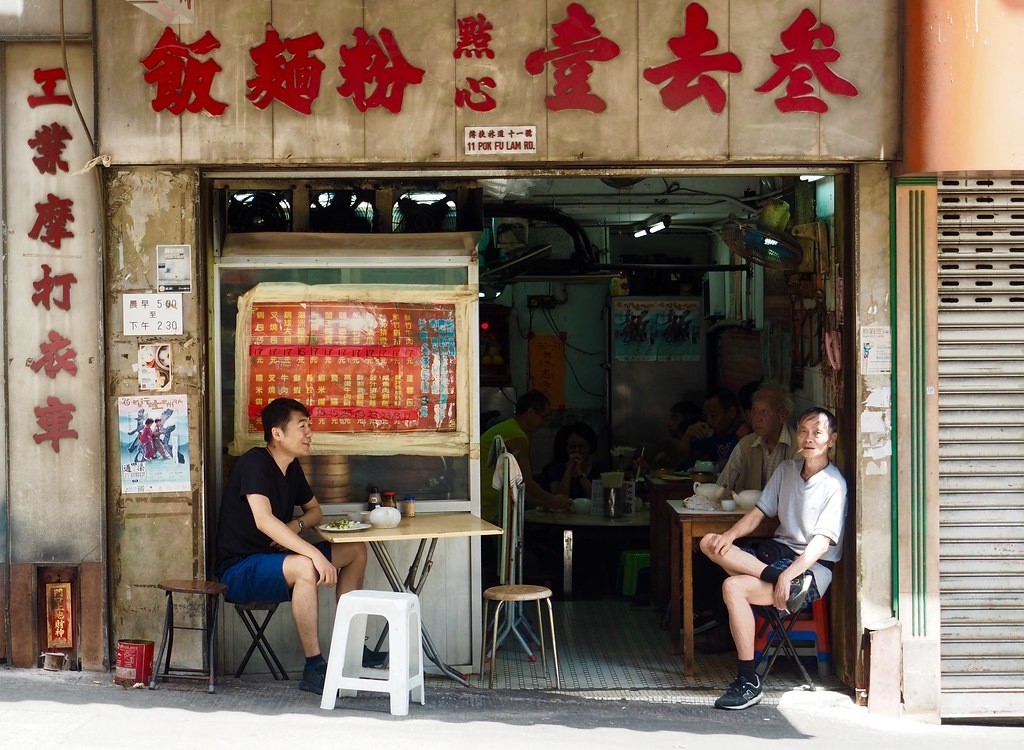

[648,465,675,478]
[689,472,716,484]
[347,511,370,523]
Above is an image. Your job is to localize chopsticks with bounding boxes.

[600,472,624,488]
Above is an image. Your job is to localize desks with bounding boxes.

[666,498,782,676]
[312,512,504,688]
[524,501,651,603]
[643,472,721,618]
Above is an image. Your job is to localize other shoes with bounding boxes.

[362,637,388,668]
[298,660,339,696]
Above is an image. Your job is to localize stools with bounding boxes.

[148,579,228,695]
[320,589,425,716]
[479,585,562,691]
[616,550,651,596]
[751,593,832,692]
[234,602,290,681]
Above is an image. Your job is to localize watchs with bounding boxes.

[295,518,304,534]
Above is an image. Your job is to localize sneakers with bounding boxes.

[678,611,718,635]
[786,574,812,612]
[714,674,763,710]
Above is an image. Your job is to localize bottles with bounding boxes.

[368,487,382,511]
[403,496,416,517]
[713,304,721,323]
[383,491,397,509]
[609,270,630,296]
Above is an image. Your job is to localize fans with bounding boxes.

[719,194,831,275]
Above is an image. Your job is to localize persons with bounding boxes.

[141,417,167,460]
[216,398,388,694]
[632,380,762,625]
[700,408,849,709]
[696,388,798,652]
[481,390,569,589]
[543,422,609,592]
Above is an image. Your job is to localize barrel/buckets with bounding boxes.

[115,639,155,686]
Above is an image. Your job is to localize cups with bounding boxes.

[603,487,625,518]
[43,652,65,671]
[721,500,736,512]
[705,429,714,437]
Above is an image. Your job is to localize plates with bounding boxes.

[662,476,692,483]
[319,523,371,532]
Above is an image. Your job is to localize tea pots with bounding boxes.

[693,460,722,474]
[369,507,402,529]
[567,498,592,514]
[693,481,728,504]
[730,489,762,510]
